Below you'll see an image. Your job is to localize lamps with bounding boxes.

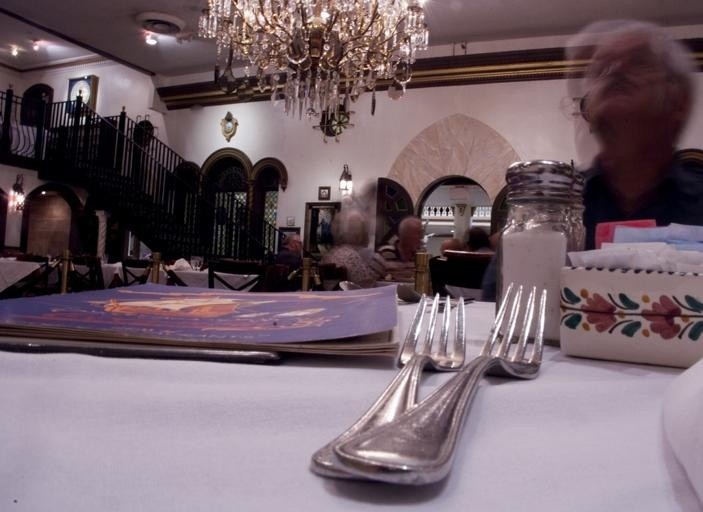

[338,164,355,199]
[196,0,431,144]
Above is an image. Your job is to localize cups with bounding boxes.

[191,255,203,272]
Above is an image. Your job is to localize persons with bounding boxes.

[260,214,501,292]
[482,19,703,304]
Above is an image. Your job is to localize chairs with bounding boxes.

[16,253,277,297]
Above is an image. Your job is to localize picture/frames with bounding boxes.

[318,186,331,200]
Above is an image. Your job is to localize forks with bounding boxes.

[309,291,465,483]
[333,282,547,488]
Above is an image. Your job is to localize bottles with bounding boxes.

[494,159,596,345]
